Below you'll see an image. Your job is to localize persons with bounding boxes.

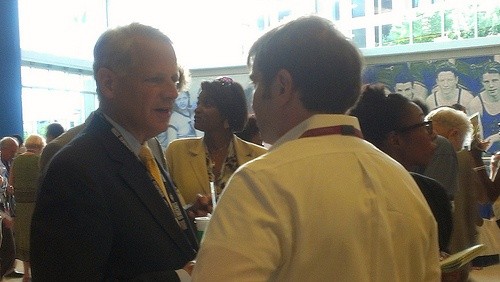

[470,61,500,219]
[29,22,213,282]
[393,72,425,102]
[234,112,265,147]
[426,65,474,115]
[159,75,268,206]
[37,62,186,195]
[347,83,500,282]
[0,123,66,282]
[191,15,440,282]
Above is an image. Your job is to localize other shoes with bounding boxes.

[5,271,24,278]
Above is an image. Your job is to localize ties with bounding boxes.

[139,146,176,213]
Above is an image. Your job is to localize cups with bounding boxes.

[194,217,210,241]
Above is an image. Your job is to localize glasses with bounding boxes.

[217,77,232,87]
[406,121,433,134]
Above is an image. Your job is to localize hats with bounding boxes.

[25,136,44,145]
